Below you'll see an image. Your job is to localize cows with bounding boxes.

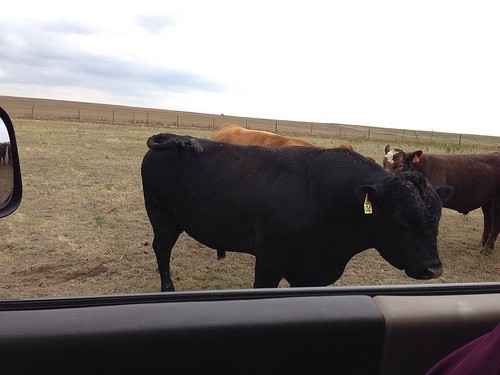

[383,143,500,254]
[209,122,353,149]
[141,133,443,290]
